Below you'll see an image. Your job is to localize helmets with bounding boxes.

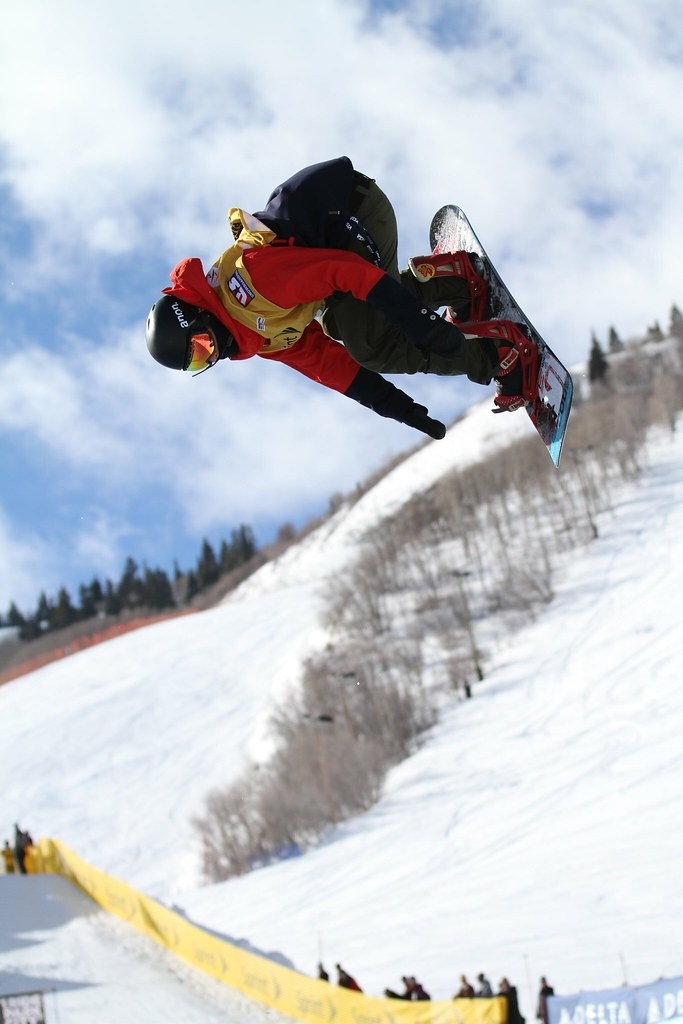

[152,295,237,369]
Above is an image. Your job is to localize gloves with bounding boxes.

[402,402,446,440]
[418,316,464,361]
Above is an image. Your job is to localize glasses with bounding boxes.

[183,331,214,371]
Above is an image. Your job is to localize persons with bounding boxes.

[458,975,474,999]
[1,841,15,874]
[499,979,518,1006]
[541,977,554,996]
[478,974,493,997]
[317,963,361,991]
[145,156,531,440]
[401,976,430,1000]
[13,824,33,874]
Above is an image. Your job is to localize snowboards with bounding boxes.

[428,203,574,472]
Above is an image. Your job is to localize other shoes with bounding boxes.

[448,257,484,322]
[492,342,531,411]
